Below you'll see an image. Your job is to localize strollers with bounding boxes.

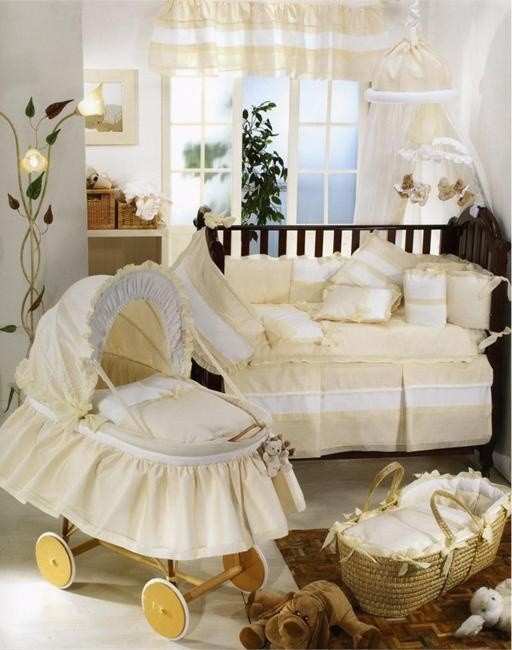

[0,262,306,641]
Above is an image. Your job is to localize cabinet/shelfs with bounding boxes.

[89,222,169,278]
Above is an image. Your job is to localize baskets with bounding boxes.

[320,462,511,618]
[117,199,160,229]
[86,189,116,229]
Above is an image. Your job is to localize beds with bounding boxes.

[193,203,510,478]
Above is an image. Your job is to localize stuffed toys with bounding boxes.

[263,440,293,477]
[394,172,474,210]
[454,578,511,639]
[238,578,382,650]
[271,432,296,456]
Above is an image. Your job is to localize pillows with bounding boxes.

[329,232,417,312]
[258,304,328,347]
[315,284,399,323]
[402,269,446,328]
[222,255,293,304]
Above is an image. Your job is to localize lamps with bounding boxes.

[0,84,108,406]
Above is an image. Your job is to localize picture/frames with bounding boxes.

[81,69,138,146]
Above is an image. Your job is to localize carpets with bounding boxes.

[276,519,511,650]
